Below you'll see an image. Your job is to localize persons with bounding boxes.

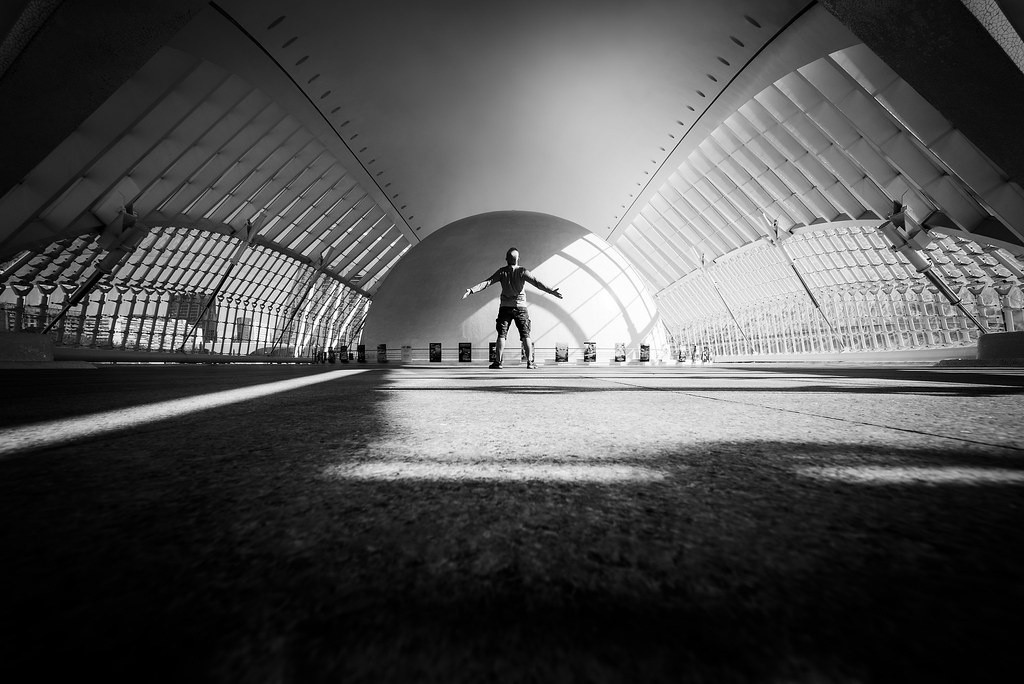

[461,247,564,368]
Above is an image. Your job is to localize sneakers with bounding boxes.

[489,359,503,369]
[527,360,538,369]
